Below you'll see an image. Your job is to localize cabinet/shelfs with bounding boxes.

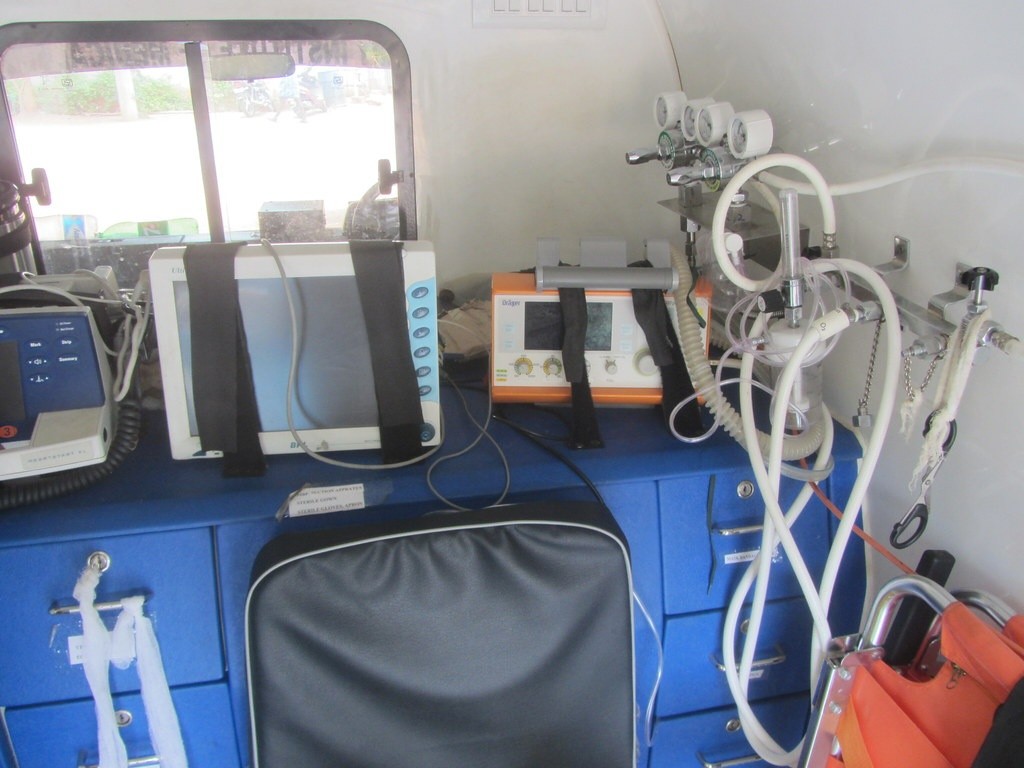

[0,340,870,768]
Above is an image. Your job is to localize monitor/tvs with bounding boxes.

[150,237,442,459]
